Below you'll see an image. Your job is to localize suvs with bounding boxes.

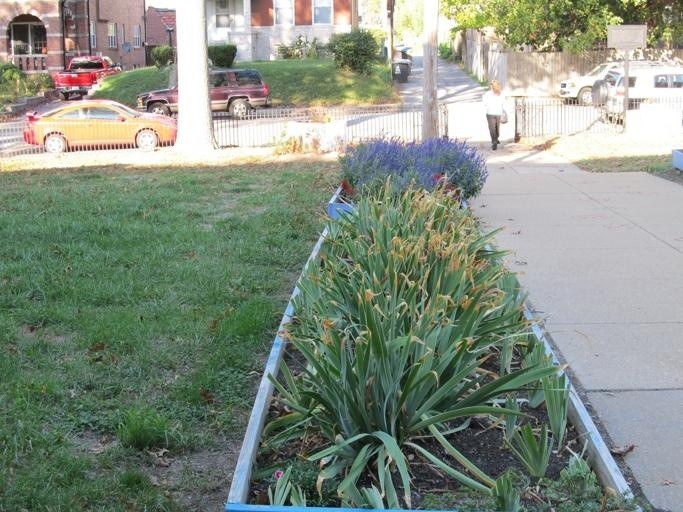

[590,62,683,112]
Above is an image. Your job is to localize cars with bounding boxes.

[20,98,177,153]
[135,68,271,120]
[558,62,622,106]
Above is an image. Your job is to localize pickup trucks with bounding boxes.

[49,55,123,100]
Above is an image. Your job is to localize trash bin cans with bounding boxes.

[392,63,409,83]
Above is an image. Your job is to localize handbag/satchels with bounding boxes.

[500,110,508,124]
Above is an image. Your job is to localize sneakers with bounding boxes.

[492,138,499,150]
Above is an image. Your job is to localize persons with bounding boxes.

[65,34,75,67]
[482,79,505,150]
